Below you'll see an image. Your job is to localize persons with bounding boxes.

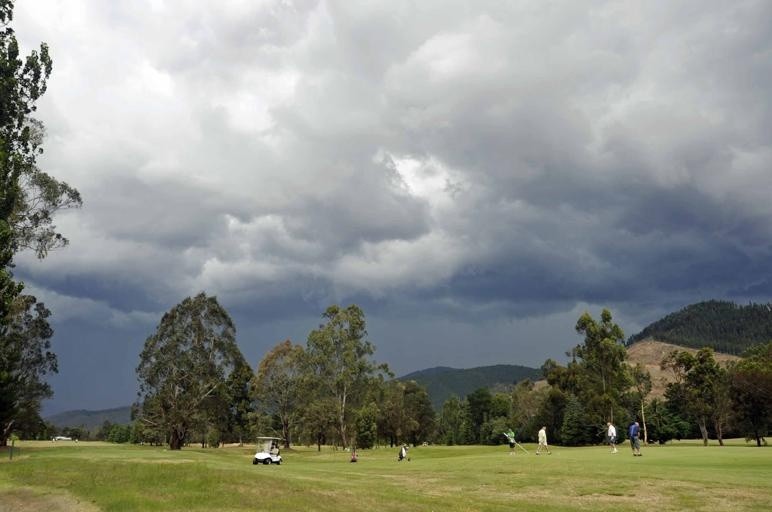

[506,428,517,456]
[606,421,618,454]
[269,445,279,456]
[627,421,643,457]
[396,445,411,463]
[535,426,552,456]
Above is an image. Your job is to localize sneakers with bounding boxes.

[632,453,641,456]
